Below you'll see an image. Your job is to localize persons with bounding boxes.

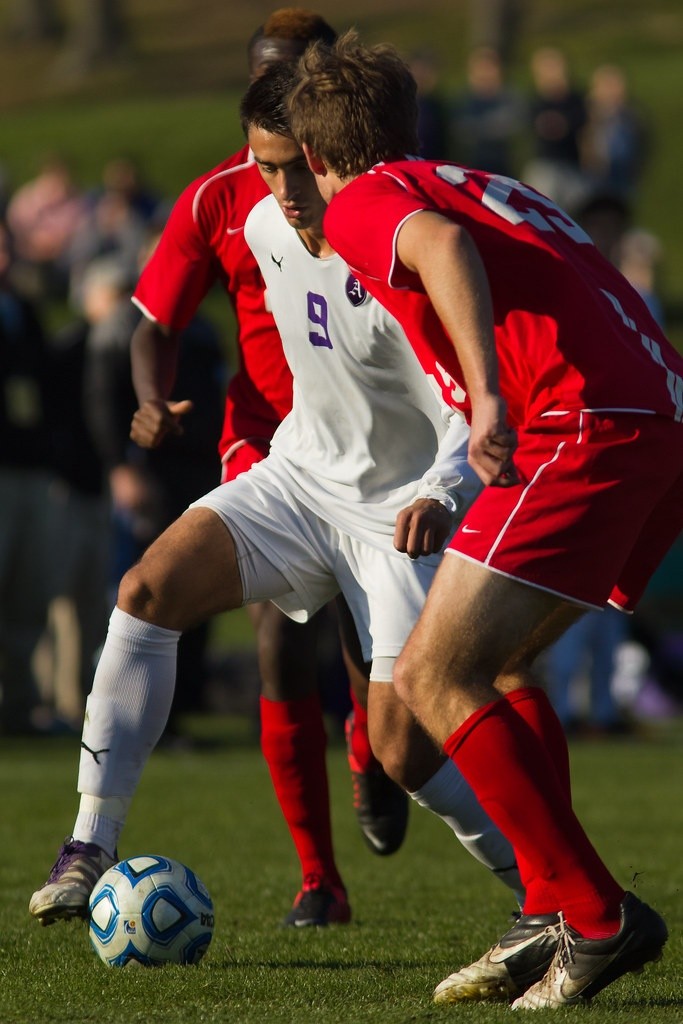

[28,64,653,981]
[286,31,683,1010]
[0,0,683,752]
[128,11,407,925]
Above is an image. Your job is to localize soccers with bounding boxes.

[85,853,216,969]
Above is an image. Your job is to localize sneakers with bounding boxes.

[287,873,351,929]
[28,836,121,927]
[430,910,572,1008]
[346,713,409,857]
[508,892,668,1012]
